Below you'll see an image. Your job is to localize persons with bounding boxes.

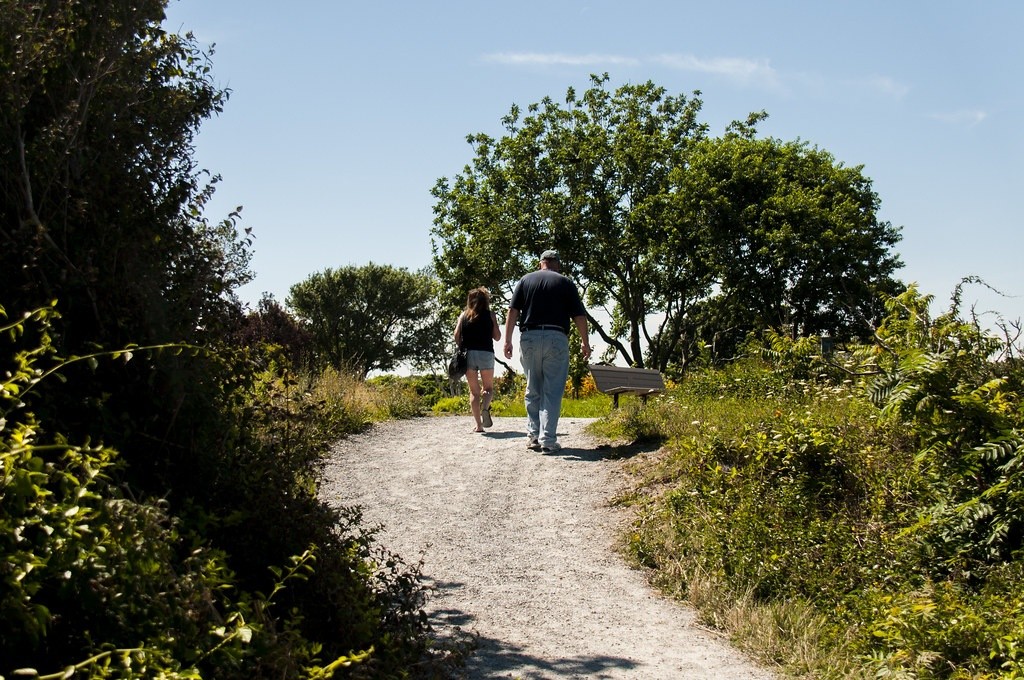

[457,287,501,433]
[504,251,590,456]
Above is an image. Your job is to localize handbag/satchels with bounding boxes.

[449,349,467,380]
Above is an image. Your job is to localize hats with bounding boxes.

[541,249,559,260]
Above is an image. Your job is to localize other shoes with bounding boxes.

[544,443,561,454]
[525,439,540,449]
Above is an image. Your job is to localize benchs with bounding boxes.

[588,365,666,408]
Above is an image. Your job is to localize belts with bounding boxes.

[521,326,568,336]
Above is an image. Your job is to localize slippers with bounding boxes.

[482,409,492,427]
